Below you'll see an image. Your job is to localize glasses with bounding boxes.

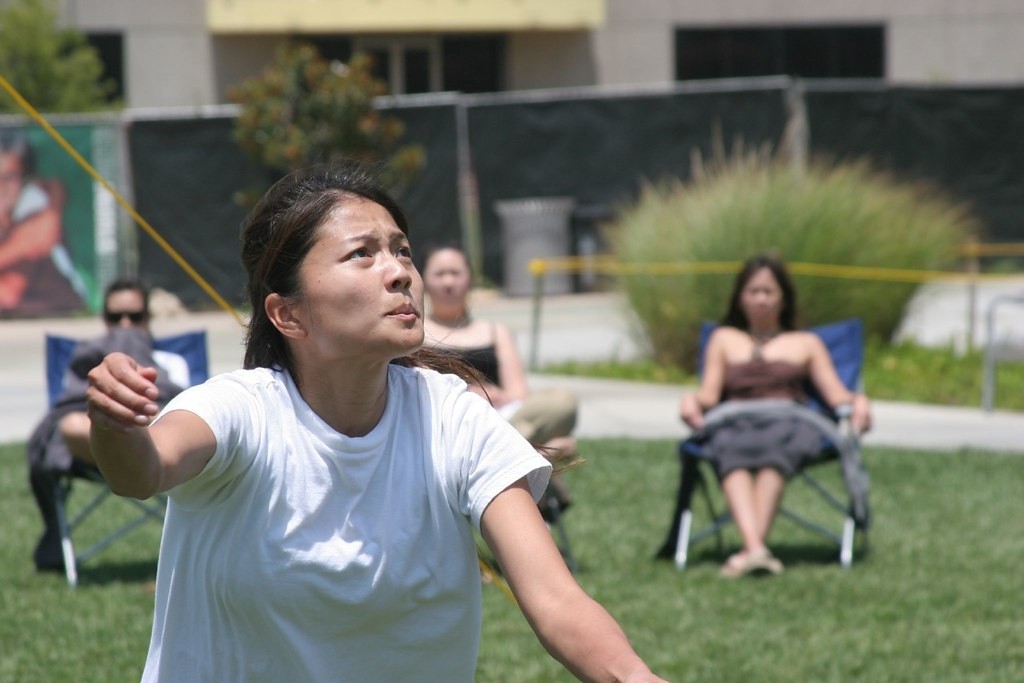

[105,309,145,323]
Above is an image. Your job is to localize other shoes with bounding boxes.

[35,529,65,570]
[720,547,785,579]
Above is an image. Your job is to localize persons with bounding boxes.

[680,257,874,576]
[60,278,190,469]
[405,245,580,450]
[87,161,670,683]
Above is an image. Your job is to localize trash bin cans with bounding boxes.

[490,195,580,295]
[572,206,619,290]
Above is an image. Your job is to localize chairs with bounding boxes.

[29,331,207,588]
[657,318,865,571]
